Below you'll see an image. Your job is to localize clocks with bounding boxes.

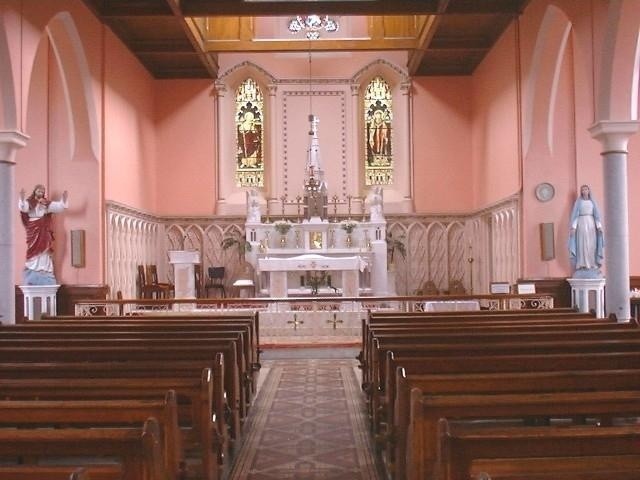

[535,182,554,202]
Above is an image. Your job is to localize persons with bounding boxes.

[567,183,605,270]
[368,111,392,158]
[238,113,260,166]
[16,183,71,285]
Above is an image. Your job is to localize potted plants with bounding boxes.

[386,231,407,270]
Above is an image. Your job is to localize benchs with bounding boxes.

[355,308,638,479]
[0,311,263,480]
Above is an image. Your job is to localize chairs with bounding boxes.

[138,265,170,299]
[195,265,200,298]
[205,267,224,299]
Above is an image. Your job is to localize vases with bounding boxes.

[344,234,353,247]
[280,234,287,248]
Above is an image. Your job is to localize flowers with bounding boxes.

[339,220,359,234]
[272,221,291,235]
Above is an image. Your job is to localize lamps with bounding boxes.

[287,14,339,40]
[70,229,86,268]
[304,41,320,195]
[540,222,555,261]
